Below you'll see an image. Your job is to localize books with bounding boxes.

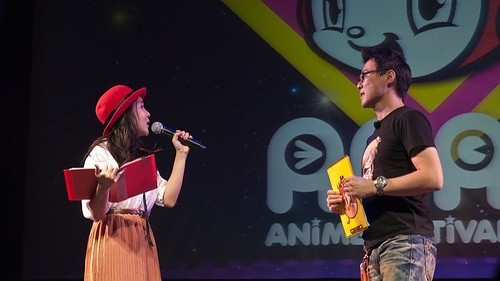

[63,155,158,203]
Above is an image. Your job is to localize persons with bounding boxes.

[327,49,443,281]
[79,84,193,281]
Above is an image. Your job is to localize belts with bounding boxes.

[106,209,145,217]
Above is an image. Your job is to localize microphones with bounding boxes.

[373,122,380,129]
[151,122,206,151]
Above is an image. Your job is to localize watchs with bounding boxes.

[373,176,388,195]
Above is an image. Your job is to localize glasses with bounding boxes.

[359,69,382,83]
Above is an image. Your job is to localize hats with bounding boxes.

[95,85,146,136]
